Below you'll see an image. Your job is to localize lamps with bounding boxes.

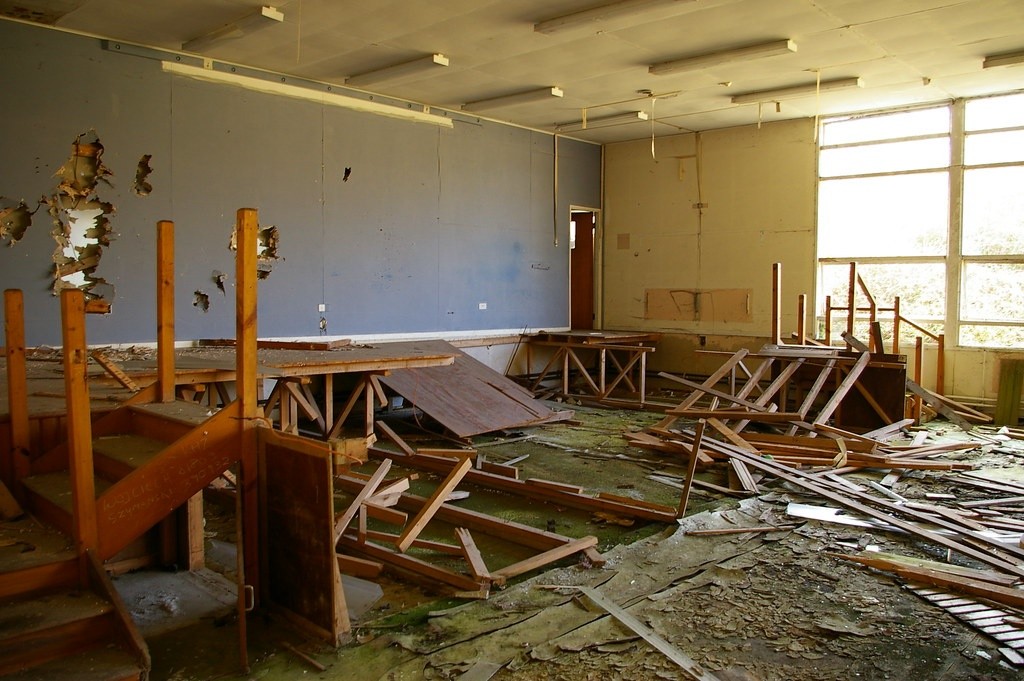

[461,88,562,111]
[983,51,1024,70]
[534,0,697,36]
[554,112,647,131]
[649,39,797,75]
[183,6,283,53]
[731,77,865,104]
[345,54,449,87]
